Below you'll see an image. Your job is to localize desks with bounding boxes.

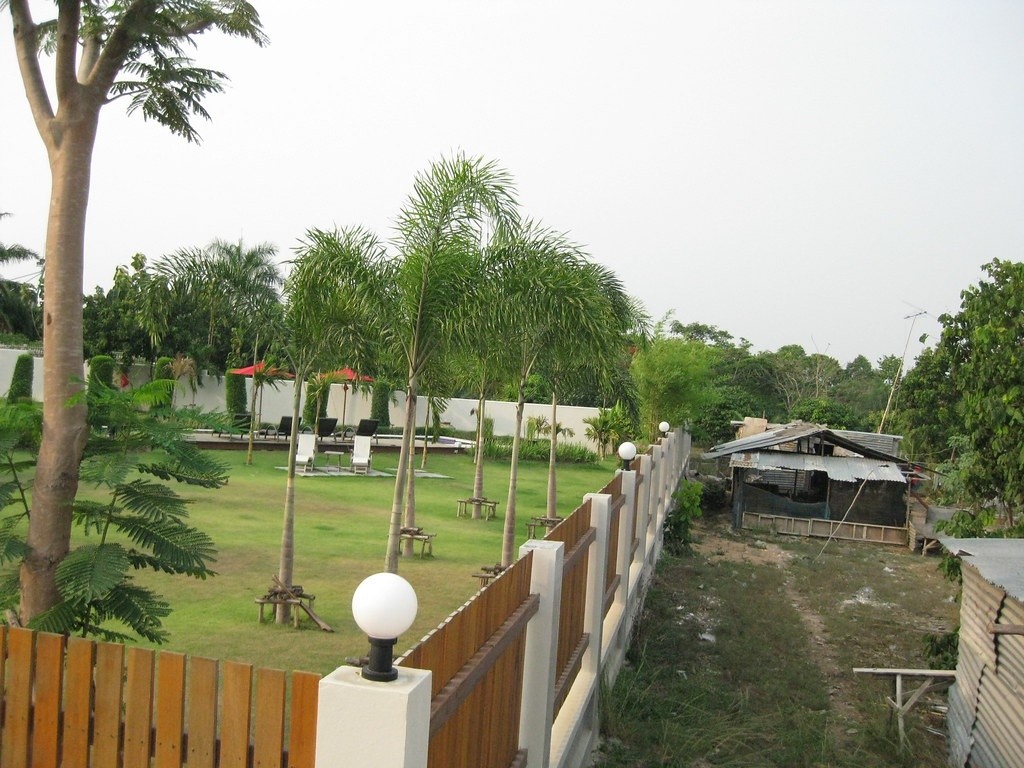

[324,451,344,473]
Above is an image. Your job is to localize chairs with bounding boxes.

[301,418,338,442]
[333,419,380,444]
[211,413,252,441]
[255,415,302,441]
[295,433,317,473]
[345,434,373,474]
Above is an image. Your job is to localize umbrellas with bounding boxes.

[311,365,375,436]
[228,359,297,440]
